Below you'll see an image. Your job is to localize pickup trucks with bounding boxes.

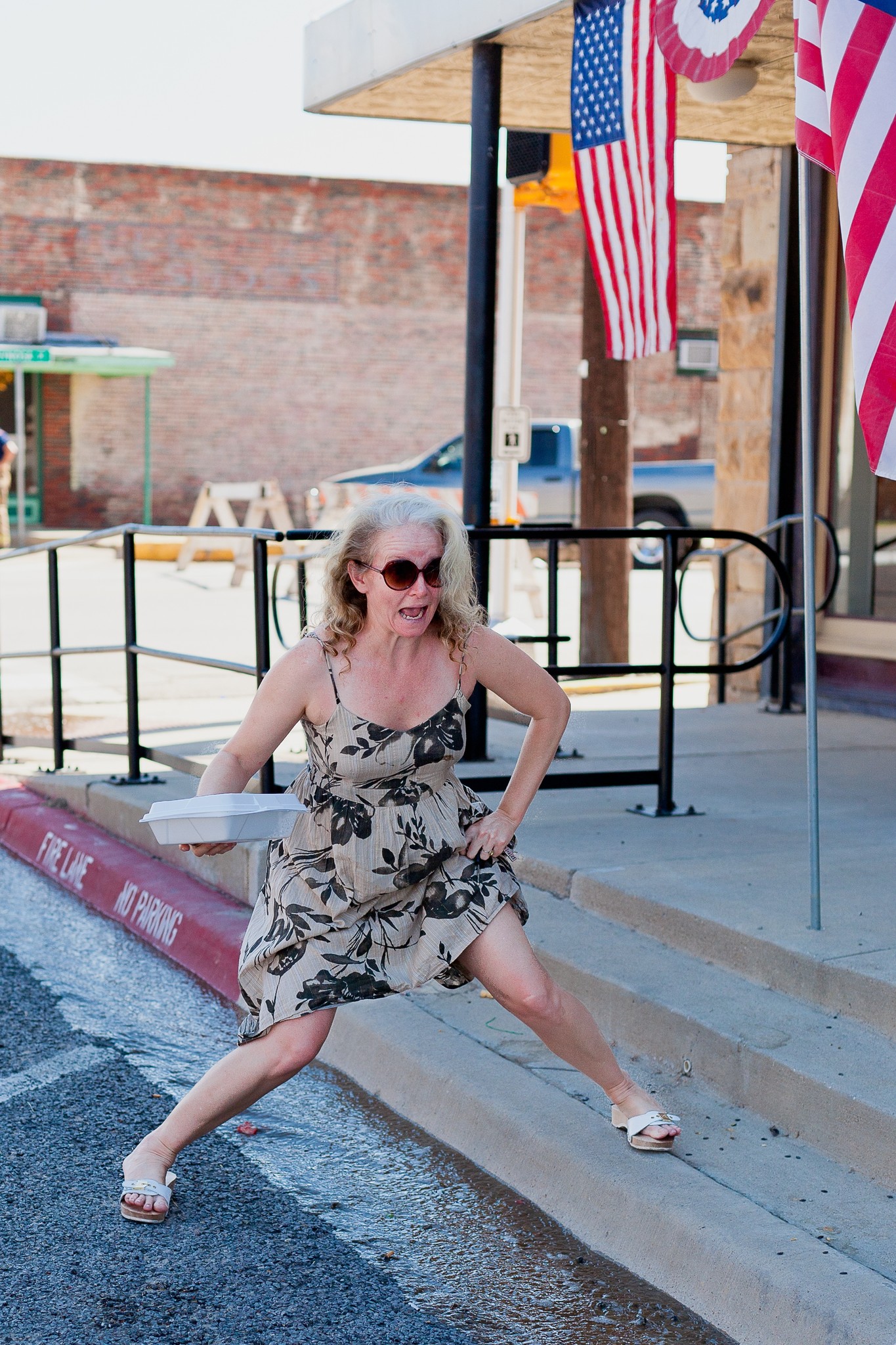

[314,419,718,573]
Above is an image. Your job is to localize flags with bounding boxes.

[792,0,896,483]
[570,0,681,361]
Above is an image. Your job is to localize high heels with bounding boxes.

[120,1171,177,1224]
[610,1102,682,1150]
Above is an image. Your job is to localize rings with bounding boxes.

[482,849,493,856]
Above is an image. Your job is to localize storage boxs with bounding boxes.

[135,786,309,851]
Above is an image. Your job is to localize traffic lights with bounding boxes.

[503,128,580,213]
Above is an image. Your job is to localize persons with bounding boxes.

[118,491,682,1226]
[0,428,18,551]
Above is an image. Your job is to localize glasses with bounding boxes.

[349,556,448,591]
